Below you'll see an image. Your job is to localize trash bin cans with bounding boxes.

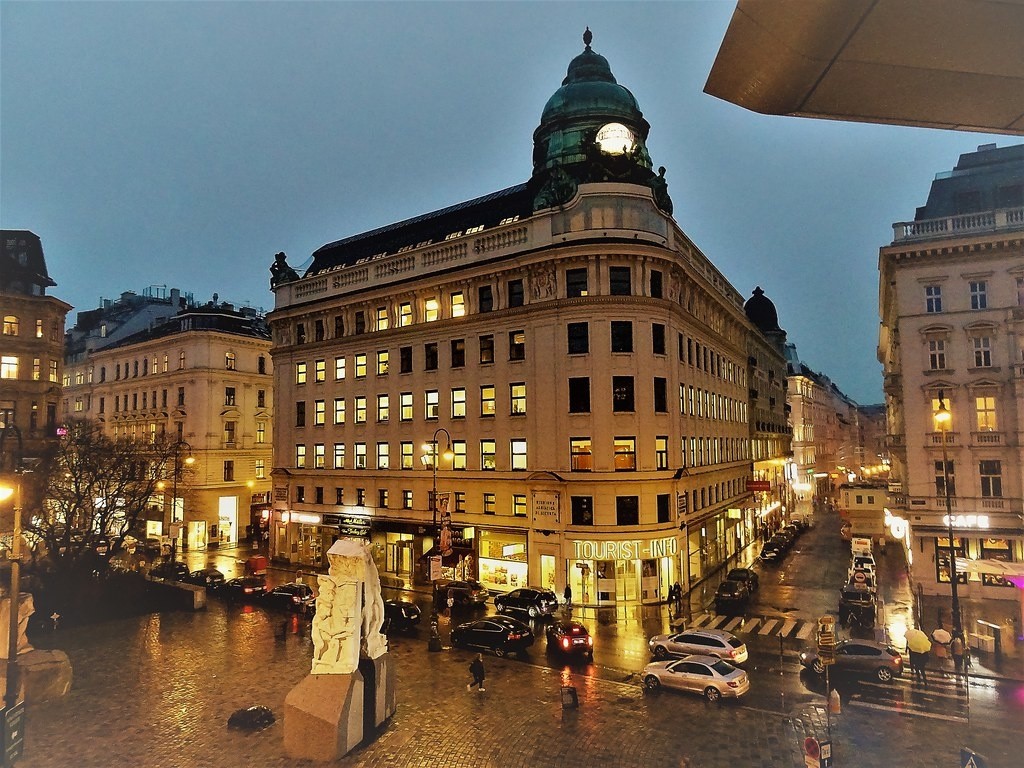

[979,635,995,653]
[561,687,579,709]
[969,633,983,648]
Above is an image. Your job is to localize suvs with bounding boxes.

[431,577,490,610]
[494,585,558,619]
[648,626,749,666]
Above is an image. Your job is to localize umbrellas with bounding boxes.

[932,629,952,644]
[904,629,932,654]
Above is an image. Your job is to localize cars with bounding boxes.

[260,583,315,615]
[218,576,267,606]
[718,567,759,599]
[148,561,190,582]
[640,654,751,704]
[799,637,904,684]
[838,533,878,630]
[450,614,535,659]
[545,620,595,662]
[381,598,422,629]
[44,524,162,558]
[182,568,225,594]
[760,519,803,563]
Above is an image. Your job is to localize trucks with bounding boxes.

[840,517,884,543]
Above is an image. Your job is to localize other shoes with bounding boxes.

[467,685,471,692]
[479,688,485,691]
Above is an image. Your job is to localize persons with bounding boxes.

[562,584,571,607]
[668,582,682,612]
[650,166,673,215]
[296,570,302,583]
[912,652,928,680]
[935,642,946,670]
[270,252,289,290]
[812,494,837,512]
[905,640,917,674]
[951,623,965,670]
[630,144,654,167]
[466,652,485,691]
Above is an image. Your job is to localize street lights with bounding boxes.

[171,441,196,581]
[934,401,966,665]
[427,427,455,652]
